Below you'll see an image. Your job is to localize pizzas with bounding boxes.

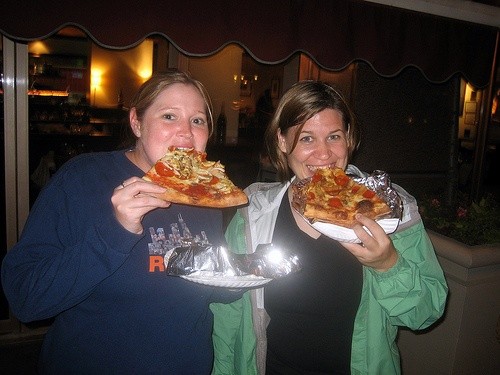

[138,146,249,208]
[304,166,390,224]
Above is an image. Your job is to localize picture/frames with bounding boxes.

[270,78,280,100]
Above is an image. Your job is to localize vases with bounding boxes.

[394,227,500,375]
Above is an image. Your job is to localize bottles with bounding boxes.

[118,88,123,109]
[217,104,226,142]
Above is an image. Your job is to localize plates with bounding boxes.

[291,206,399,243]
[163,246,273,287]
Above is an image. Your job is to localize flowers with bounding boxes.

[414,189,500,247]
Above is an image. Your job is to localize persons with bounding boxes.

[256,88,275,140]
[210,79,450,375]
[0,66,247,375]
